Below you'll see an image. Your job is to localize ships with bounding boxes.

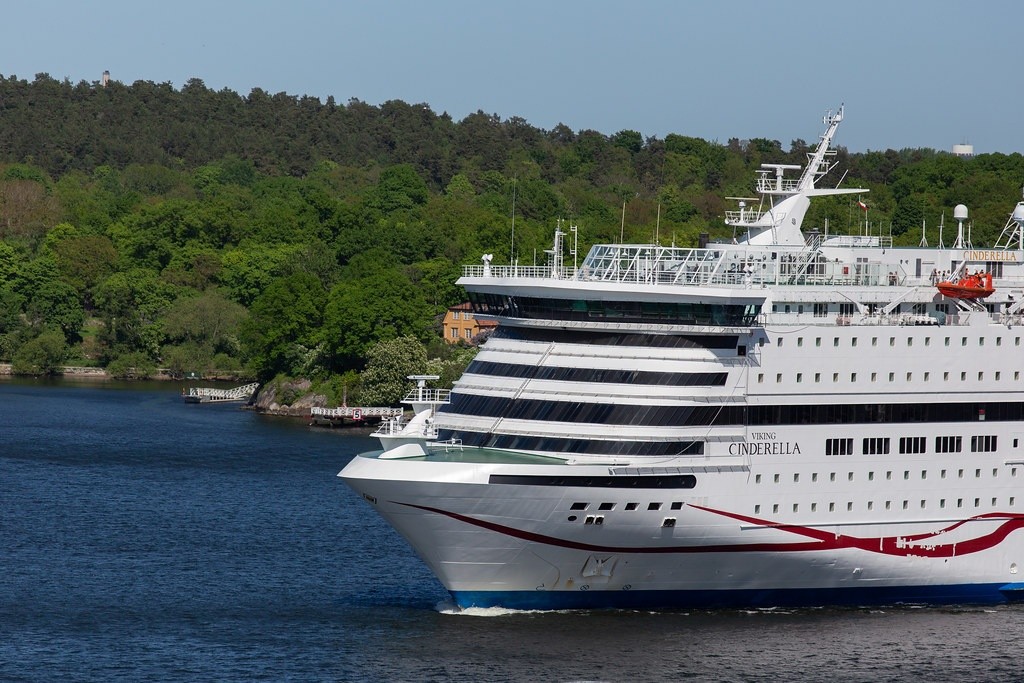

[335,96,1024,611]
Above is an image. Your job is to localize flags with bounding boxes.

[859,196,869,211]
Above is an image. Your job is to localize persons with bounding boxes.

[930,268,984,286]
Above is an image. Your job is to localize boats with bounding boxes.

[935,272,996,299]
